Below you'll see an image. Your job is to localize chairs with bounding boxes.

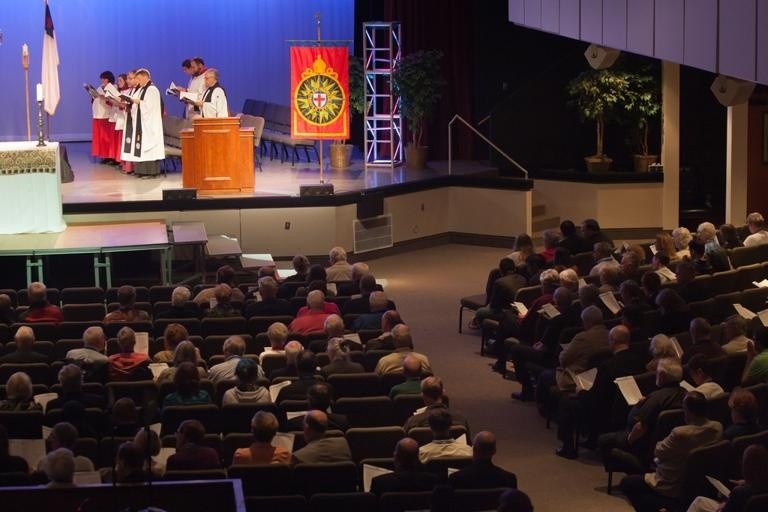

[162,99,323,177]
[0,223,768,512]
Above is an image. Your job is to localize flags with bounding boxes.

[291,45,351,142]
[40,4,62,118]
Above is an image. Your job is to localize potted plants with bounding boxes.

[392,53,445,171]
[620,72,662,175]
[563,69,630,174]
[329,56,370,168]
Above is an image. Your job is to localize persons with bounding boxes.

[0,247,472,509]
[83,55,228,182]
[448,430,519,488]
[475,213,766,508]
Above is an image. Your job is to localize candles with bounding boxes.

[36,83,44,101]
